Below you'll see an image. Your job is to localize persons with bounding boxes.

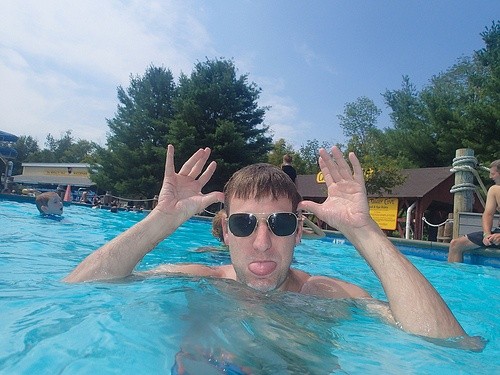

[446,159,500,263]
[59,139,472,340]
[280,153,299,191]
[35,191,63,216]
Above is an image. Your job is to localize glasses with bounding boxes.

[226,212,301,238]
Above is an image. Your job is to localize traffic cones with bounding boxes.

[63,184,72,203]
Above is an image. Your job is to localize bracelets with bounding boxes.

[482,232,491,237]
